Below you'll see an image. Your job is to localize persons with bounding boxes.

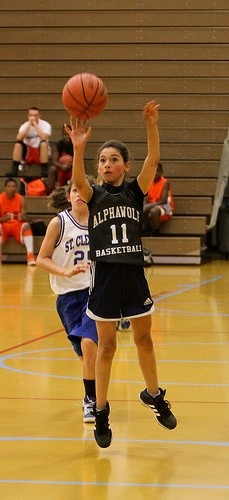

[36,175,98,423]
[64,100,178,448]
[5,107,52,178]
[143,162,175,237]
[48,125,74,188]
[0,179,36,266]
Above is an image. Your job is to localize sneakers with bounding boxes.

[138,387,177,430]
[89,400,112,448]
[81,397,95,423]
[115,319,130,331]
[40,168,47,177]
[4,169,18,177]
[26,254,36,266]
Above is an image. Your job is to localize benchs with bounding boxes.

[0,0,229,264]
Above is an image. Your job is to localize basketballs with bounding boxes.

[62,73,108,120]
